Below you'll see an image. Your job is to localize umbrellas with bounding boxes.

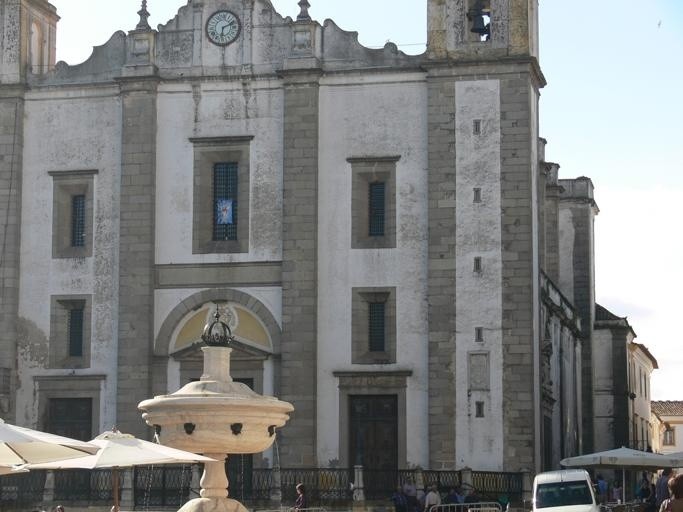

[558,444,683,504]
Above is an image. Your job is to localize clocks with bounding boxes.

[205,10,241,47]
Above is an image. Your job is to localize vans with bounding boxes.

[531,469,599,512]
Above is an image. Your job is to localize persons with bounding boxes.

[447,488,459,503]
[634,479,657,512]
[659,472,683,512]
[595,473,609,502]
[611,479,623,502]
[294,483,310,512]
[456,488,465,503]
[424,484,441,512]
[465,489,481,502]
[403,476,417,508]
[656,468,673,510]
